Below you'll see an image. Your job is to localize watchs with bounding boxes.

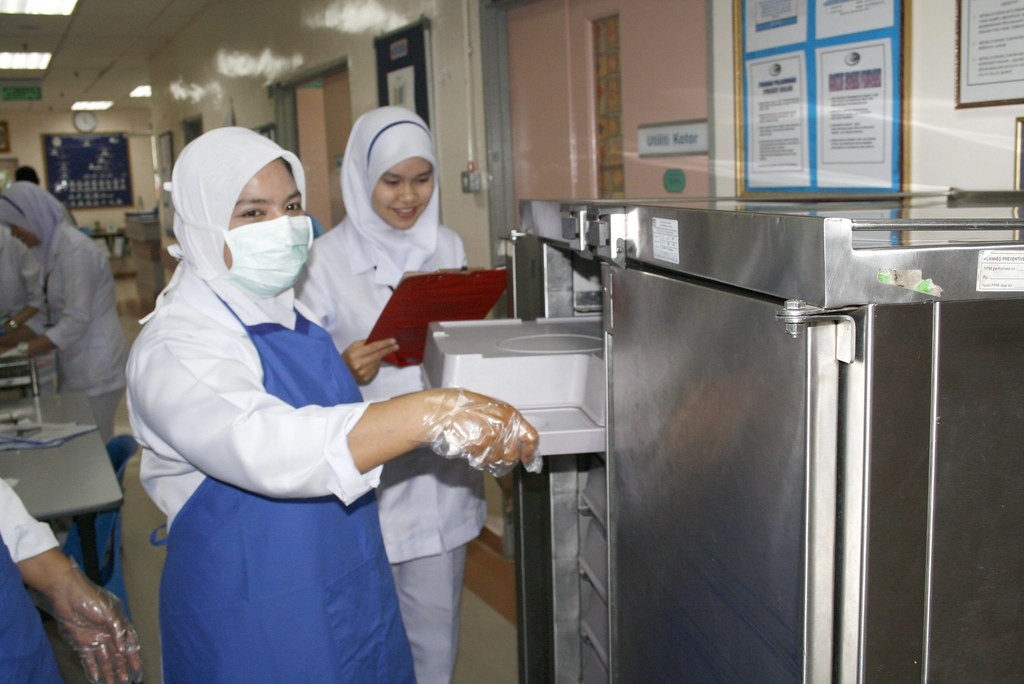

[8,318,18,329]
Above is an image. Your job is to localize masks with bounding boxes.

[223,215,314,300]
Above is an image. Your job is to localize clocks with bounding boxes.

[73,111,97,133]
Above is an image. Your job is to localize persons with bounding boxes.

[127,125,538,684]
[294,106,487,684]
[0,165,144,684]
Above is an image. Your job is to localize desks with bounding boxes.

[91,228,125,258]
[0,389,125,593]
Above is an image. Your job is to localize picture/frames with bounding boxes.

[0,121,10,154]
